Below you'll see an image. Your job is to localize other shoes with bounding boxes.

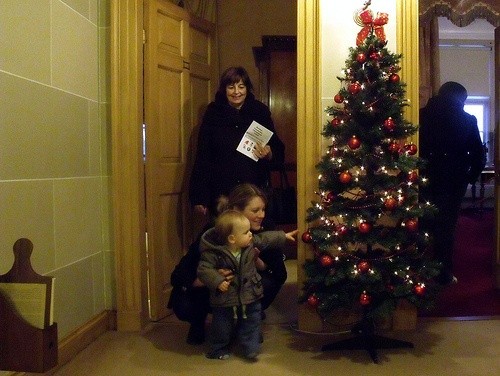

[216,354,229,360]
[245,352,257,358]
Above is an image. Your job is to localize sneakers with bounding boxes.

[187,321,206,346]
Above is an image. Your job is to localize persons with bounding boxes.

[166,184,288,346]
[419,81,486,285]
[196,212,299,362]
[187,65,285,221]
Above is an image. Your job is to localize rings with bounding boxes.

[259,151,262,154]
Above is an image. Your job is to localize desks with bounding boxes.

[472,171,496,216]
